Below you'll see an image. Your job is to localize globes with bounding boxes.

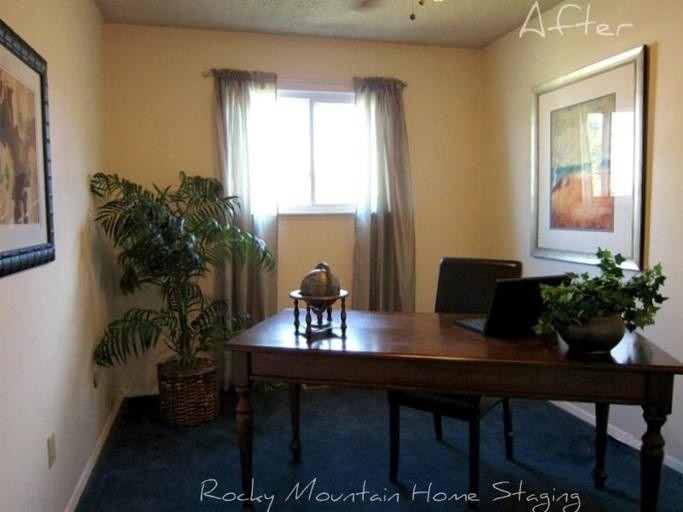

[289,262,349,339]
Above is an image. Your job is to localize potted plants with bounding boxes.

[86,171,276,424]
[530,249,668,353]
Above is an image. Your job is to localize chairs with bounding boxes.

[385,257,523,508]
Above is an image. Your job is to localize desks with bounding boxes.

[223,307,682,511]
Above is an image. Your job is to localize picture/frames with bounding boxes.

[0,20,55,278]
[529,43,648,273]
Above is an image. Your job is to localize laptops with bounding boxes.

[453,275,571,336]
